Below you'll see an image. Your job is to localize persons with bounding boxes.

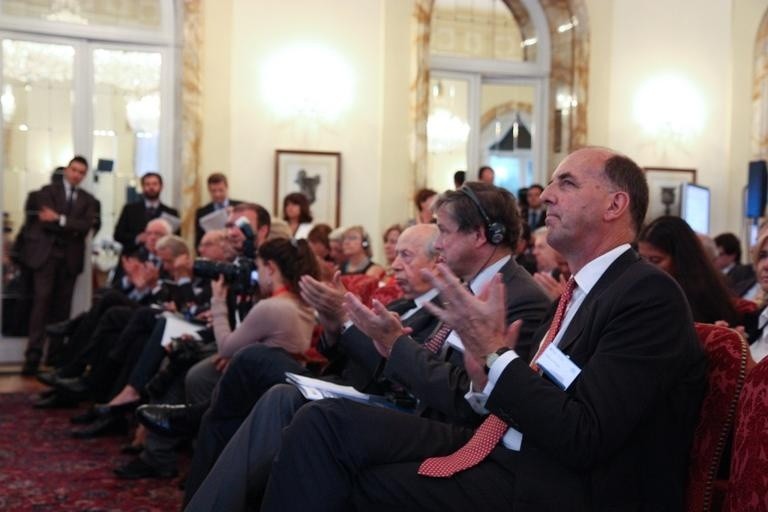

[9,143,768,512]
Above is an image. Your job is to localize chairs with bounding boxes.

[298,272,408,383]
[681,296,767,511]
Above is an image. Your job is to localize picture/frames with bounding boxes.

[271,146,342,237]
[640,164,698,231]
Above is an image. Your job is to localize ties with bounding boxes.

[414,274,578,480]
[425,280,474,358]
[530,211,537,224]
[66,184,77,213]
[148,206,155,220]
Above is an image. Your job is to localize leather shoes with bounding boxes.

[114,459,180,480]
[134,400,211,439]
[33,313,150,454]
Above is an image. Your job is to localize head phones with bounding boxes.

[460,184,507,245]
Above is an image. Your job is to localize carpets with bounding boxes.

[0,383,190,509]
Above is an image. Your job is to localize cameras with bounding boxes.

[193,216,259,294]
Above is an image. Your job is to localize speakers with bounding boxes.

[745,160,768,218]
